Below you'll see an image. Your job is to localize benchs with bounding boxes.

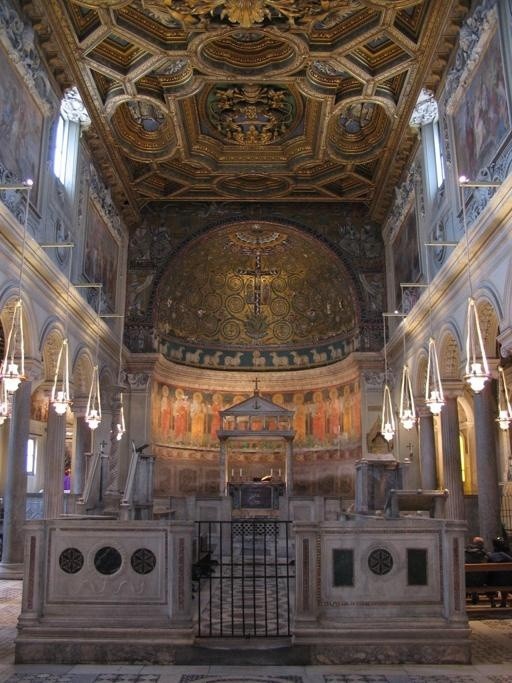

[465,563,512,618]
[192,532,219,600]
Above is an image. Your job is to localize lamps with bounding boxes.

[381,181,512,443]
[0,179,127,443]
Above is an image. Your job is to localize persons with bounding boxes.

[160,385,172,435]
[291,392,307,445]
[172,388,189,446]
[233,395,244,404]
[341,386,350,436]
[86,243,116,295]
[273,394,283,406]
[350,382,361,437]
[190,392,205,444]
[1,74,39,188]
[209,393,222,439]
[328,388,340,439]
[460,69,508,175]
[311,391,327,442]
